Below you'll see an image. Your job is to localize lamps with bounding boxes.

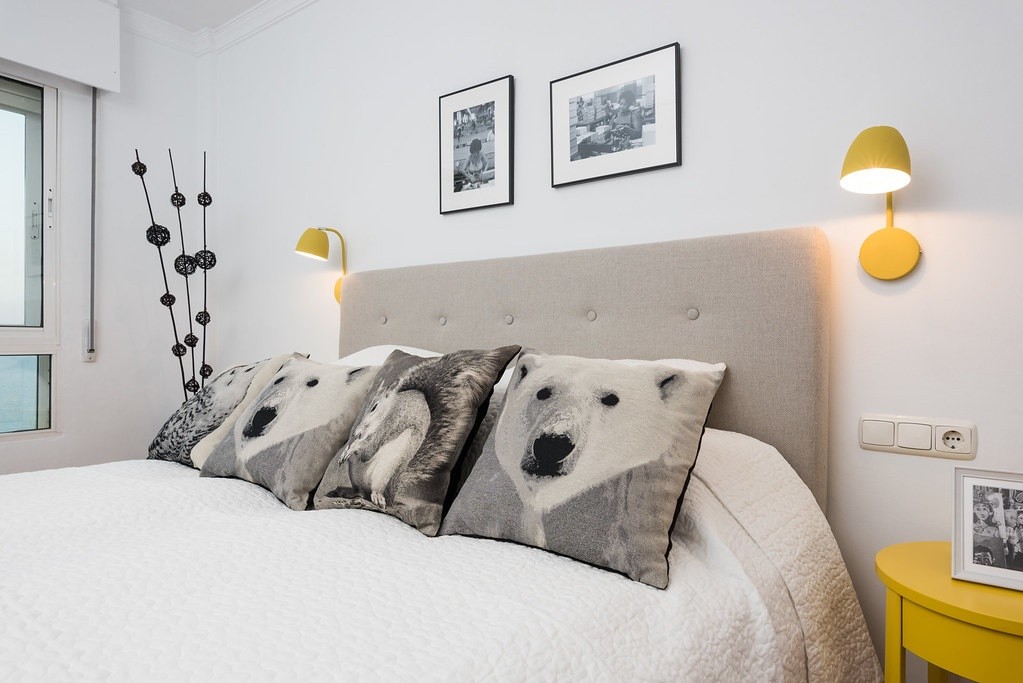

[294,227,347,304]
[838,125,920,283]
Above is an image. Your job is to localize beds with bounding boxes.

[0,226,885,683]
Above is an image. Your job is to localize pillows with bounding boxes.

[300,345,526,534]
[436,347,732,589]
[199,351,385,515]
[145,358,272,470]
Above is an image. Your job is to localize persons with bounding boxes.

[608,91,642,139]
[460,139,489,182]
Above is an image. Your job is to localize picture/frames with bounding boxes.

[549,41,680,188]
[437,75,515,215]
[949,465,1023,591]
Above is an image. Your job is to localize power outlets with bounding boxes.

[859,412,977,461]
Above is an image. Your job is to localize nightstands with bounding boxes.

[872,540,1023,683]
